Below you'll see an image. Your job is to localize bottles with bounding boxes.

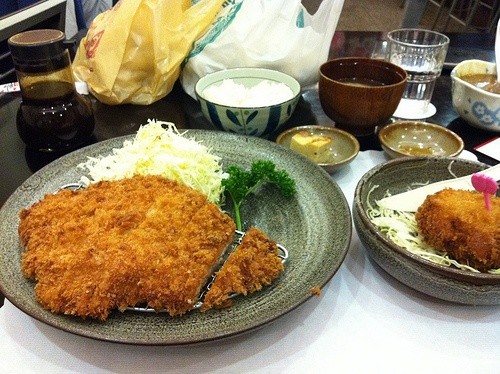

[7,29,97,151]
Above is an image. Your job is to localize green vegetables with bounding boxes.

[221,159,297,233]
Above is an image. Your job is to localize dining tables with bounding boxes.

[0,28,500,374]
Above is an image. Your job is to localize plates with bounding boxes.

[0,129,352,346]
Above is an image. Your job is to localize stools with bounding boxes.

[401,0,500,31]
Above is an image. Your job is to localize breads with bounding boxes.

[290,130,332,164]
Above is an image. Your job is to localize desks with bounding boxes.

[0,0,67,87]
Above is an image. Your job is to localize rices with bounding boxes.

[203,78,295,108]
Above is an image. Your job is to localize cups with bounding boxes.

[387,27,449,116]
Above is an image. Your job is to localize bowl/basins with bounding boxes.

[351,154,500,305]
[450,58,500,131]
[319,57,408,136]
[193,68,303,140]
[379,120,465,159]
[276,124,360,173]
